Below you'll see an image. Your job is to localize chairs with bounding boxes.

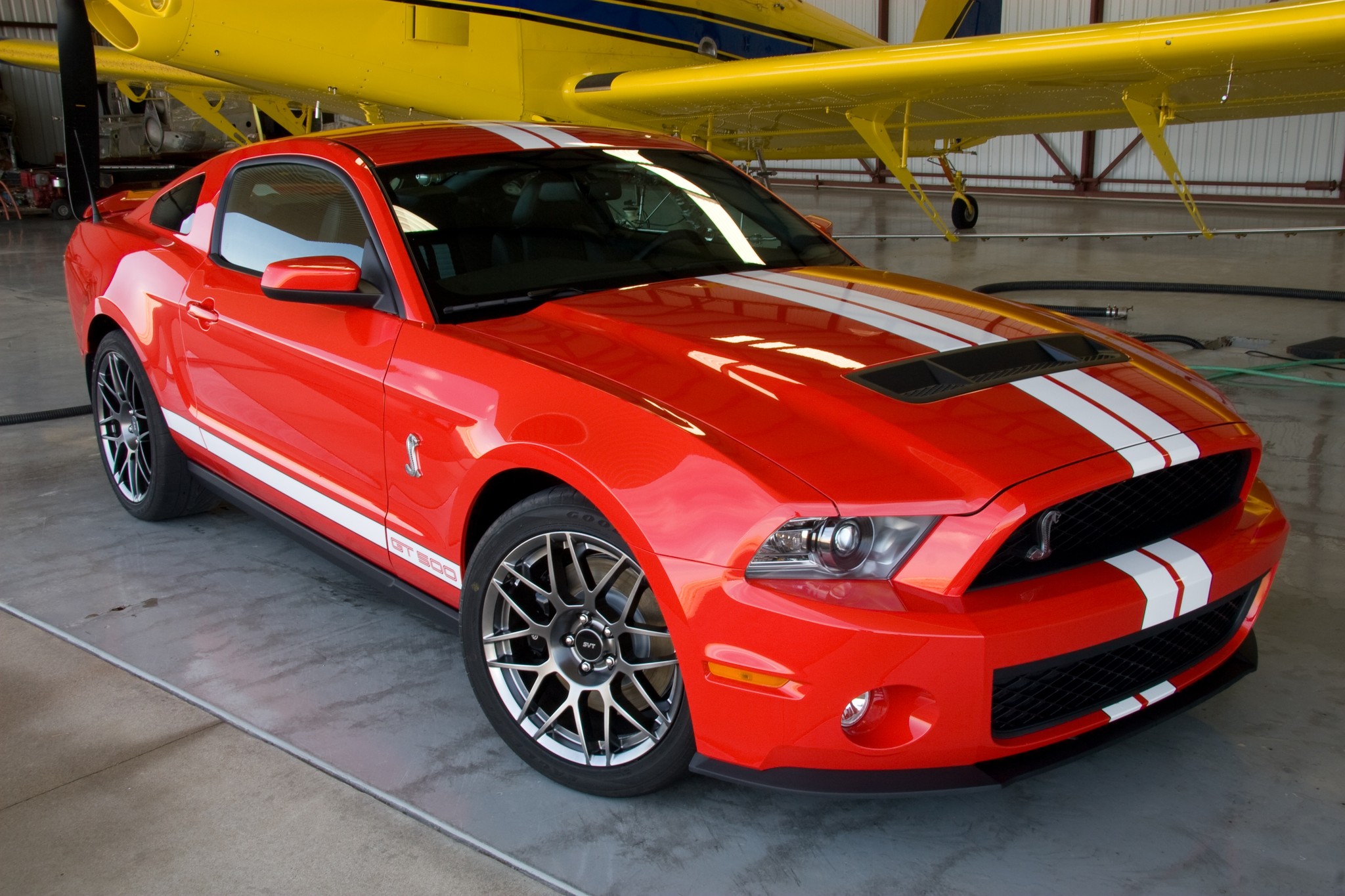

[491,172,619,268]
[395,185,471,281]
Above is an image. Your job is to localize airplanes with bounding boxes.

[0,0,1345,240]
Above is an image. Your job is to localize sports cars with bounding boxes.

[59,118,1292,801]
